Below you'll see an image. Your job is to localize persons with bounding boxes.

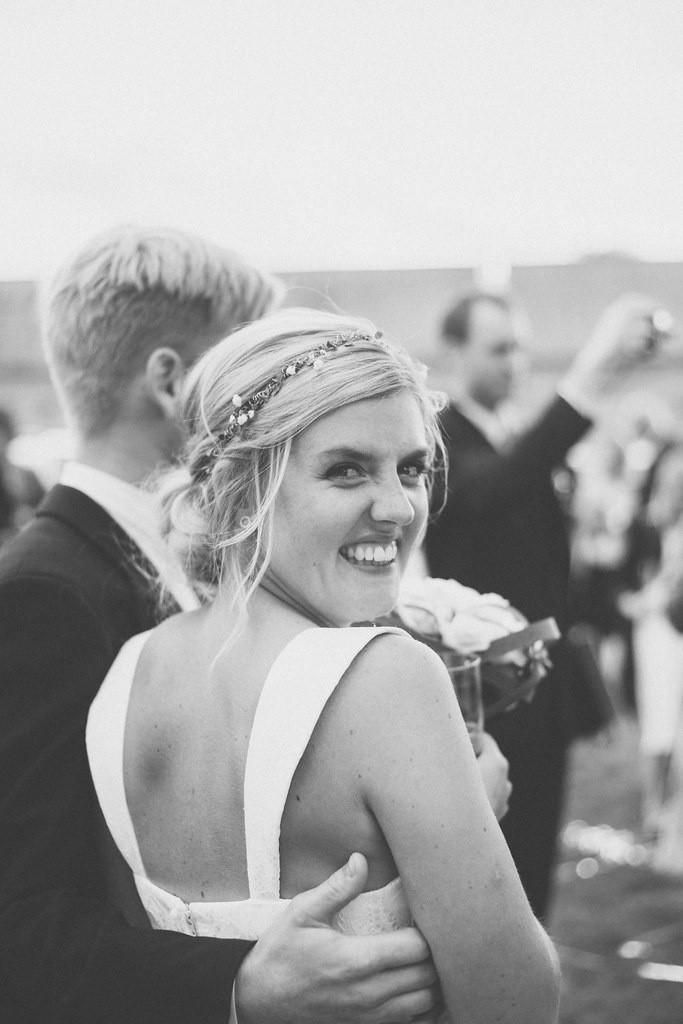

[0,412,46,545]
[422,288,663,920]
[0,226,558,1024]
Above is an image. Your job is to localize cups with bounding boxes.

[435,652,485,758]
